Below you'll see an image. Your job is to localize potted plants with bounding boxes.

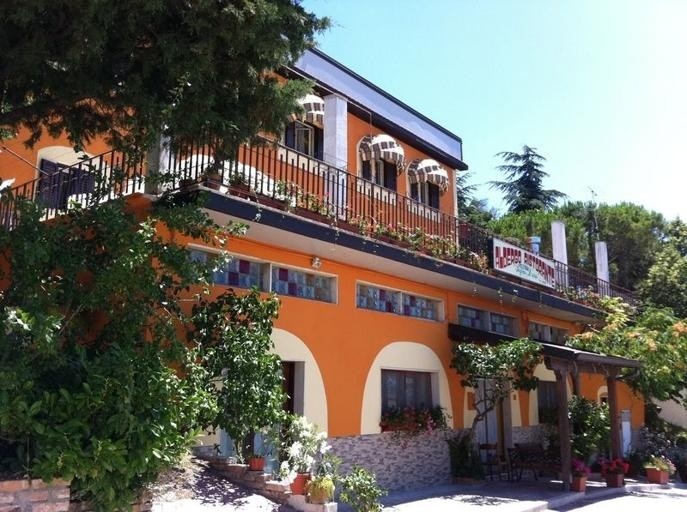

[243,413,347,503]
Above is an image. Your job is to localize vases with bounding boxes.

[645,468,670,484]
[572,476,586,491]
[606,473,624,488]
[180,173,288,210]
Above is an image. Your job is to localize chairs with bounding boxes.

[478,443,561,483]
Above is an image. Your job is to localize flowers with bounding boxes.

[642,454,677,475]
[277,177,489,272]
[598,457,629,474]
[379,403,452,435]
[572,457,590,477]
